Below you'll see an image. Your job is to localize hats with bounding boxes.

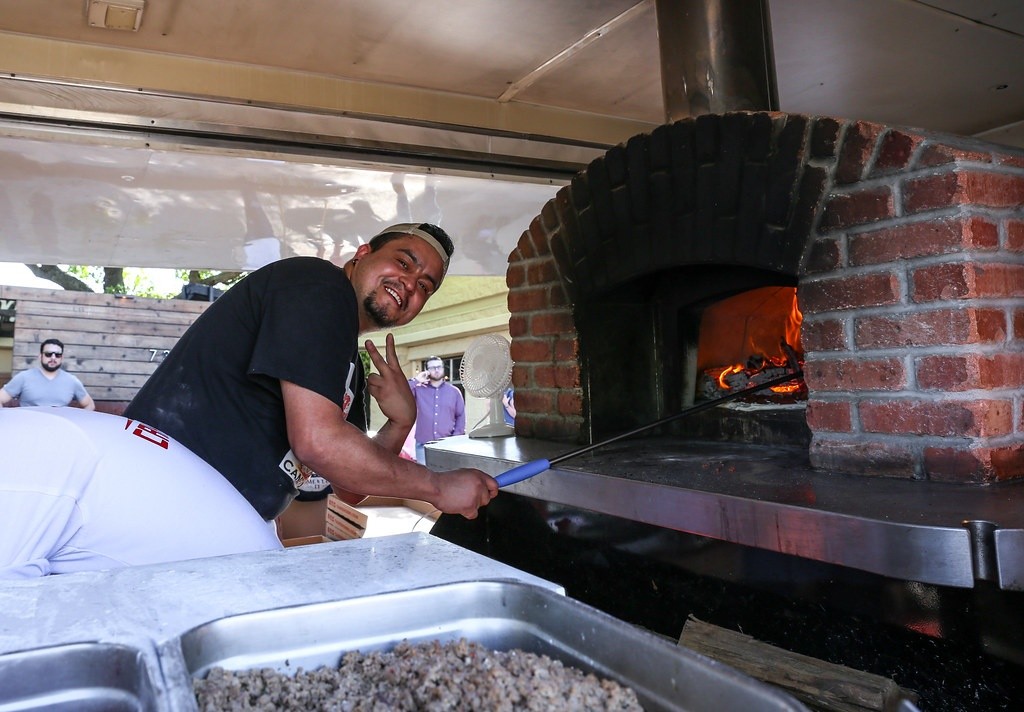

[376,222,455,294]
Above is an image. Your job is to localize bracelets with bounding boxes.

[505,405,509,408]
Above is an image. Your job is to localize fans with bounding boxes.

[459,335,516,438]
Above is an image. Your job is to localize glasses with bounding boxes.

[426,365,443,371]
[41,350,62,358]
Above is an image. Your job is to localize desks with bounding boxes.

[0,531,566,656]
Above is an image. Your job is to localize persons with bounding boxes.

[502,388,516,426]
[0,406,285,581]
[0,339,95,411]
[122,222,498,537]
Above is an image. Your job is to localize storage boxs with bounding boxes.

[278,494,442,548]
